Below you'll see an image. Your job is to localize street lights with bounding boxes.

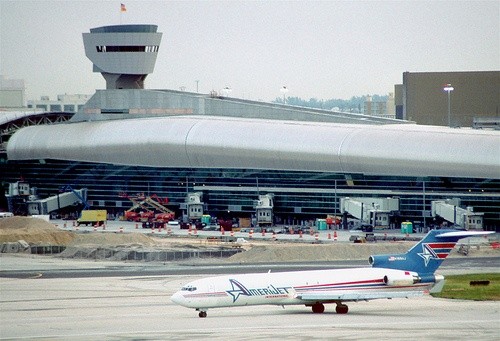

[443,86,455,127]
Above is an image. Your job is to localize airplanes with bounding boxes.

[171,227,497,318]
